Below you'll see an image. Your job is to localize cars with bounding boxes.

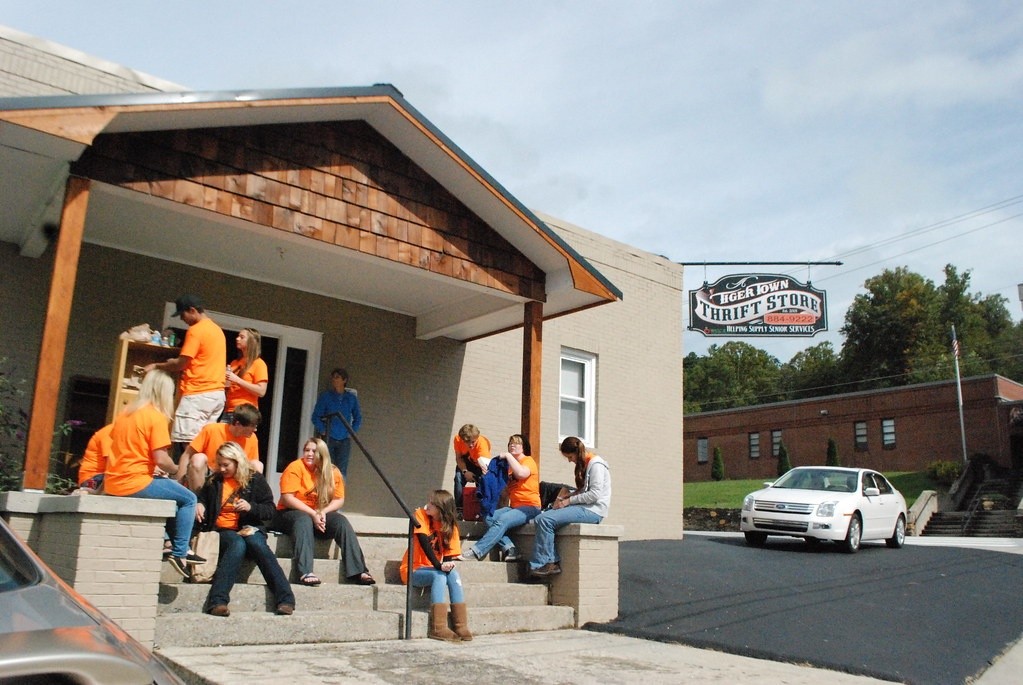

[740,466,907,553]
[0,515,183,685]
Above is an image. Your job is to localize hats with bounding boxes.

[170,293,201,318]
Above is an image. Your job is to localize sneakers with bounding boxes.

[532,563,561,576]
[457,550,479,561]
[505,546,522,561]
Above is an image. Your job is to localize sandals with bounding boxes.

[352,571,375,584]
[296,573,321,585]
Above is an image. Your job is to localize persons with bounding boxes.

[78,423,172,552]
[453,424,491,521]
[218,328,268,423]
[400,490,472,642]
[528,437,611,576]
[103,369,207,578]
[456,434,541,562]
[176,402,261,495]
[275,437,375,585]
[311,368,361,486]
[194,441,295,617]
[140,293,225,449]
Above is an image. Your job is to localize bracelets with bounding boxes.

[462,469,468,475]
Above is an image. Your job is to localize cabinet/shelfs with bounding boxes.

[55,374,110,485]
[105,339,181,425]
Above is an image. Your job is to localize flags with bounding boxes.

[951,326,958,357]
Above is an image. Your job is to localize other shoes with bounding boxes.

[211,605,230,616]
[186,550,207,563]
[456,507,463,520]
[277,604,295,615]
[168,556,191,578]
[163,544,172,553]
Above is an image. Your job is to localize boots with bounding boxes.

[429,603,461,642]
[450,602,472,641]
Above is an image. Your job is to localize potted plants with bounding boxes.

[982,495,994,511]
[990,494,1008,509]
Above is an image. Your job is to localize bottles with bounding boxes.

[225,365,232,388]
